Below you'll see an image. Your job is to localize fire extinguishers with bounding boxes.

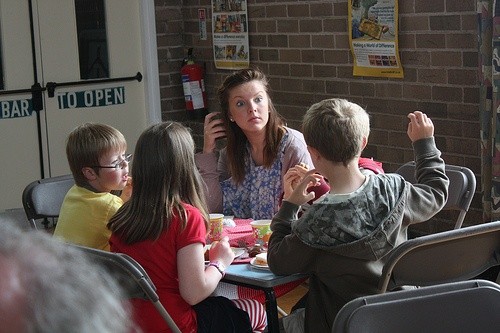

[181,47,208,111]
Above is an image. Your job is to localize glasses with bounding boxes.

[88,154,132,171]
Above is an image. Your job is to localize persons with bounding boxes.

[264,96,451,333]
[106,121,266,333]
[52,122,131,253]
[194,70,316,304]
[0,217,143,333]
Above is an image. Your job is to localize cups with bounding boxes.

[206,213,225,245]
[250,219,273,252]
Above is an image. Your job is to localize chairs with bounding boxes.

[393,160,478,230]
[373,219,500,293]
[329,279,500,333]
[21,172,78,232]
[66,243,183,333]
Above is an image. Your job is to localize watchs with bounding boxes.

[206,261,226,277]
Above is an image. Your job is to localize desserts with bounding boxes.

[255,252,267,265]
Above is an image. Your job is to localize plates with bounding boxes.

[204,244,245,263]
[250,257,270,272]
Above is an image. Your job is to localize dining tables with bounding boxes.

[204,218,312,333]
